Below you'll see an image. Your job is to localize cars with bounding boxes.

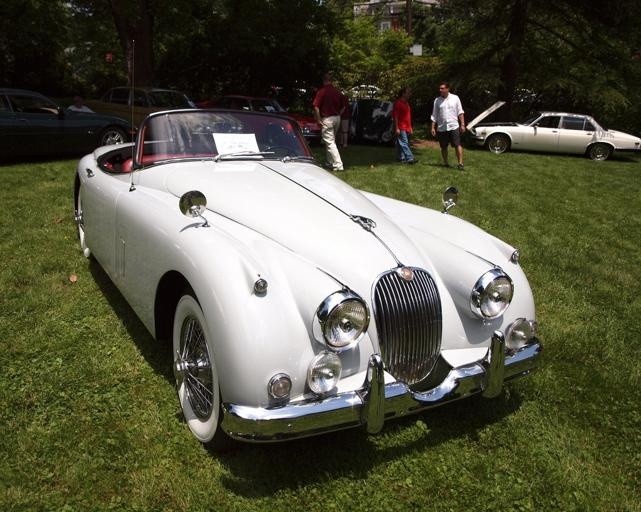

[460,99,640,163]
[354,98,401,146]
[85,89,199,127]
[0,88,138,163]
[210,95,322,144]
[70,103,555,459]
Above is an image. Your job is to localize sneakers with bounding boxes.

[457,163,463,170]
[408,160,419,164]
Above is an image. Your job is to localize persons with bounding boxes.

[391,86,419,165]
[427,81,467,171]
[335,90,353,151]
[313,72,348,174]
[67,96,95,115]
